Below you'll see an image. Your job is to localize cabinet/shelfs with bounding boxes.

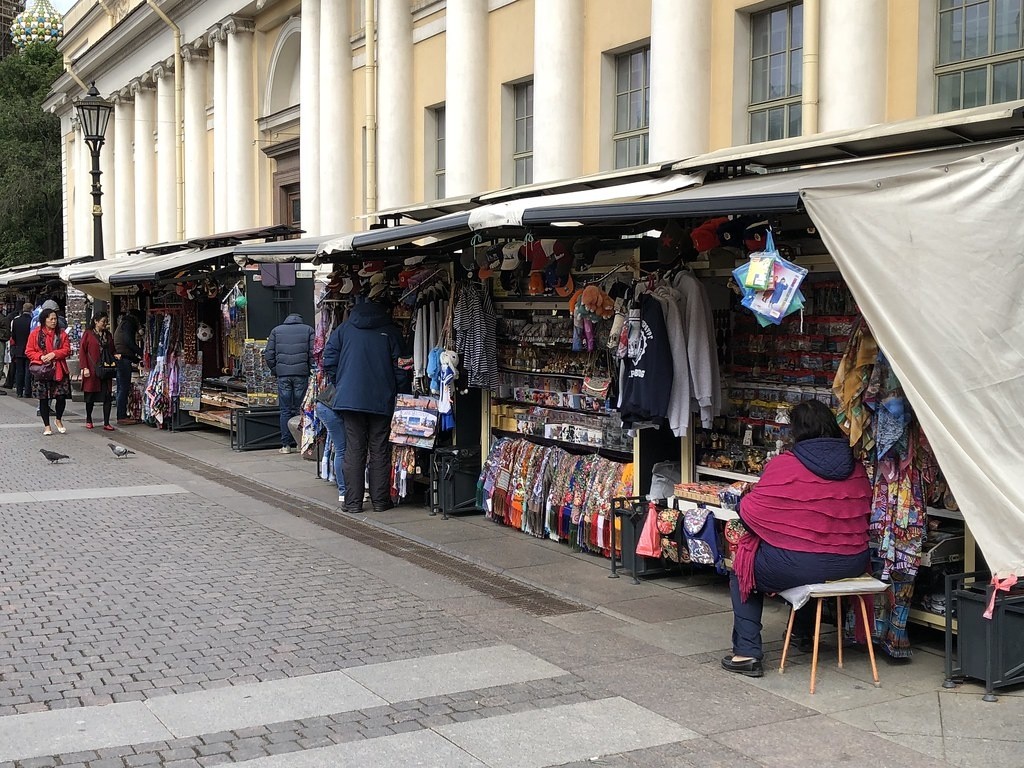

[187,377,271,432]
[483,301,652,460]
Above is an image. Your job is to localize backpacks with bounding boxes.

[656,499,752,565]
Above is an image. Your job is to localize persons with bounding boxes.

[264,313,315,454]
[6,299,69,415]
[25,309,72,434]
[313,300,401,513]
[717,398,873,675]
[78,310,122,430]
[114,307,144,423]
[0,302,8,378]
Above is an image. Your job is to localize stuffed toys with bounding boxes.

[566,285,616,329]
[428,347,444,394]
[526,273,554,297]
[438,349,459,412]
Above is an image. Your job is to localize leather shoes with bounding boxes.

[721,656,763,677]
[783,631,813,652]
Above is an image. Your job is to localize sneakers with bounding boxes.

[296,445,301,453]
[278,445,290,454]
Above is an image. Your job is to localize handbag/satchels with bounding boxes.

[388,390,440,449]
[29,361,55,382]
[582,349,618,400]
[650,460,681,499]
[96,364,117,380]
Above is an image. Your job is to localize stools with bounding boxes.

[779,590,884,694]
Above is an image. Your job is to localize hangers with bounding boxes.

[415,270,442,302]
[639,260,687,295]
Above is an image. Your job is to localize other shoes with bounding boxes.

[54,419,66,433]
[374,499,394,512]
[118,417,136,425]
[104,424,114,430]
[0,383,55,416]
[43,428,53,435]
[86,423,93,429]
[339,490,370,502]
[341,501,362,513]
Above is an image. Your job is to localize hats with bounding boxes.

[657,215,771,267]
[23,303,34,312]
[327,232,605,298]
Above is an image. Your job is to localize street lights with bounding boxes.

[74,78,114,319]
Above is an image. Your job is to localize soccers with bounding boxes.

[196,325,214,342]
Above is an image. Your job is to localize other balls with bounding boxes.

[235,295,246,308]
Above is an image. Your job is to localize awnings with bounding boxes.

[0,255,198,285]
[231,222,412,259]
[108,219,306,287]
[350,137,1023,253]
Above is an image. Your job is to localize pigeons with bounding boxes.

[38,449,70,464]
[107,443,136,459]
